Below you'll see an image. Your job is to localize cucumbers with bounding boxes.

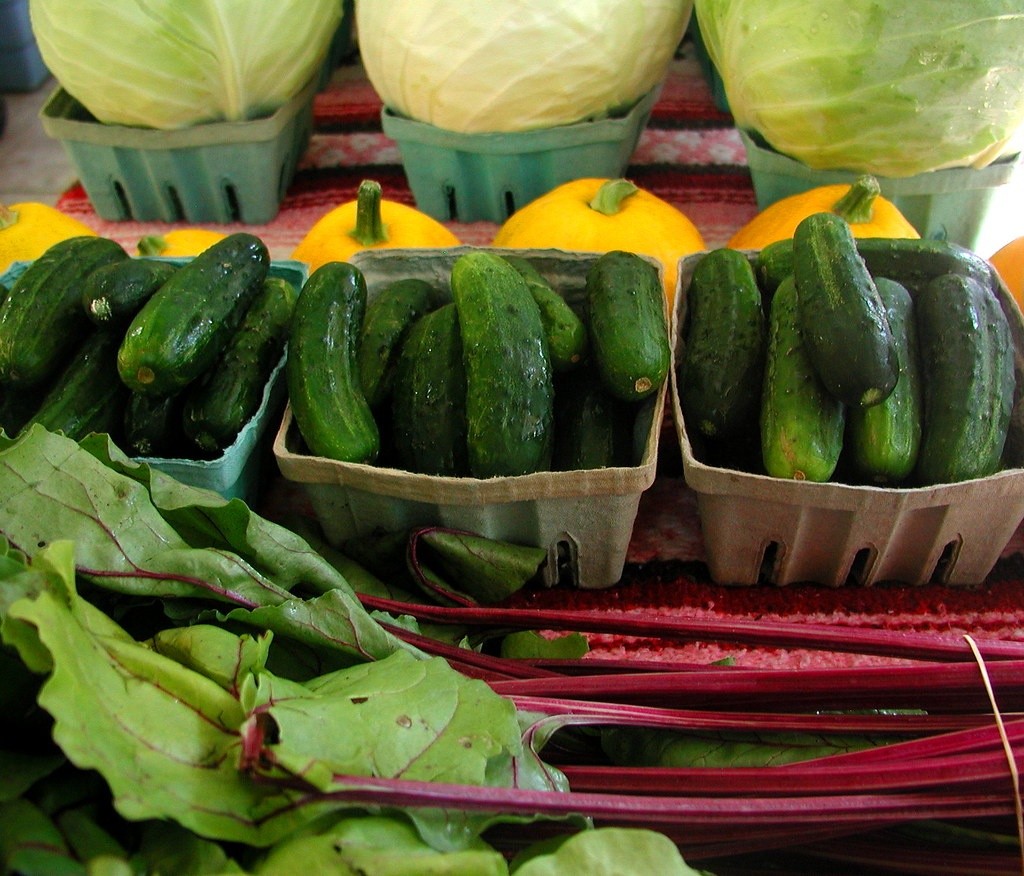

[0,211,1017,491]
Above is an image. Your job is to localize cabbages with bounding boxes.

[26,0,1023,181]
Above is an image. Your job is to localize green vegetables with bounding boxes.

[0,414,1024,875]
